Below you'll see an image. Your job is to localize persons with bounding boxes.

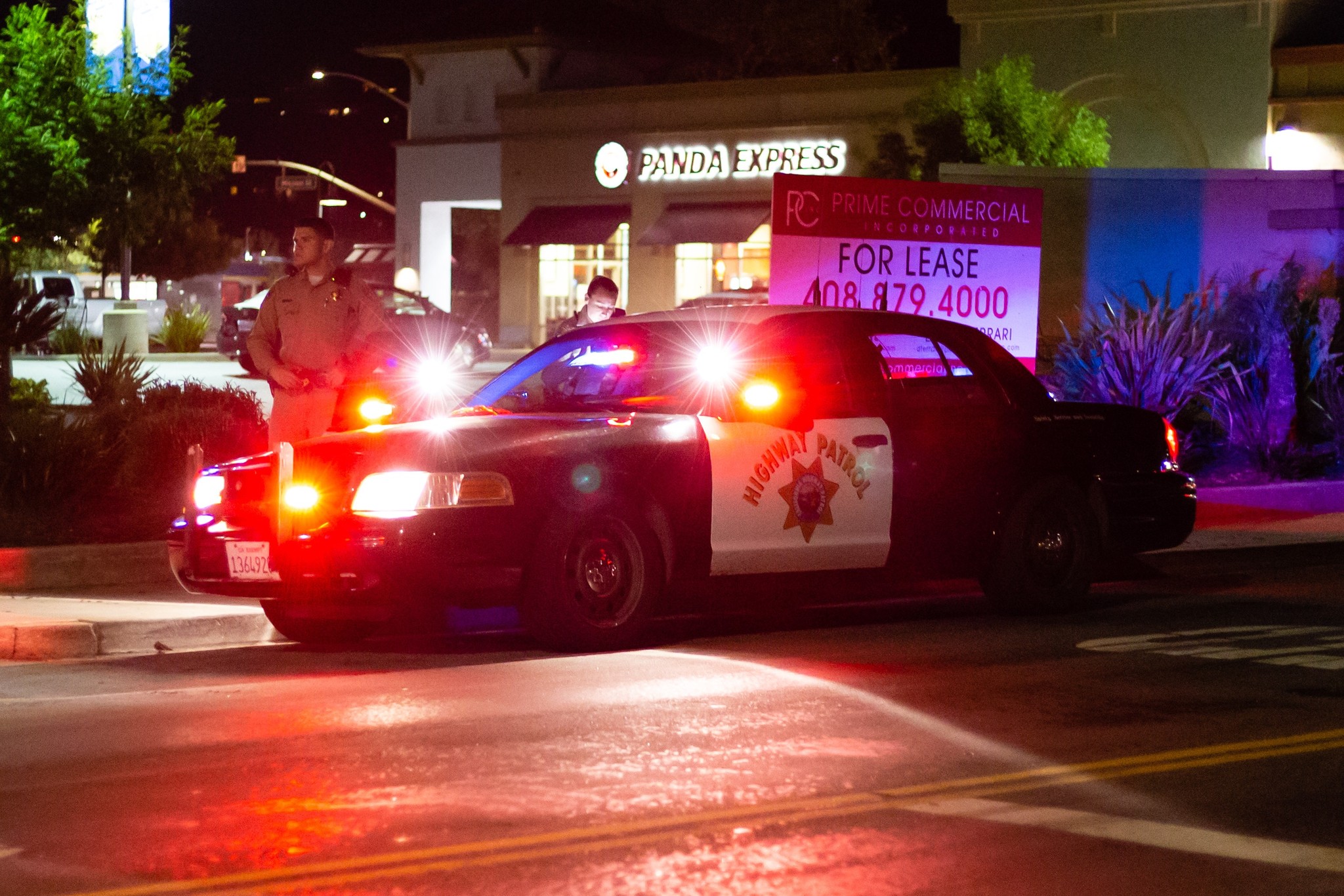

[540,275,627,410]
[244,217,385,454]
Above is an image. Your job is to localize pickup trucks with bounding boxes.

[12,272,174,349]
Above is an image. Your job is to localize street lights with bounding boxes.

[80,0,170,351]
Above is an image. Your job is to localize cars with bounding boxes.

[220,283,491,386]
[168,298,1198,650]
